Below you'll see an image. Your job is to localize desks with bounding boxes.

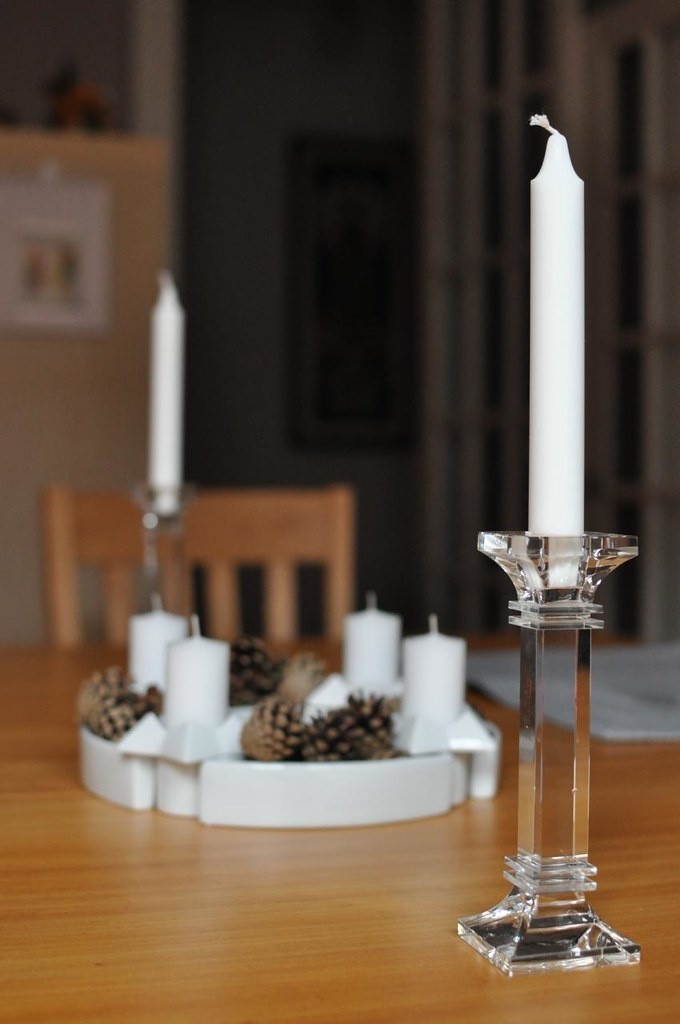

[3,635,680,1023]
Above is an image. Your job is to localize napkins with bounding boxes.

[471,637,680,742]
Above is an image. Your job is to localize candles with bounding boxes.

[528,113,586,591]
[402,615,469,751]
[165,614,228,735]
[126,593,188,694]
[343,591,402,697]
[147,264,184,514]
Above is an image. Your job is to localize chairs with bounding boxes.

[44,483,357,648]
